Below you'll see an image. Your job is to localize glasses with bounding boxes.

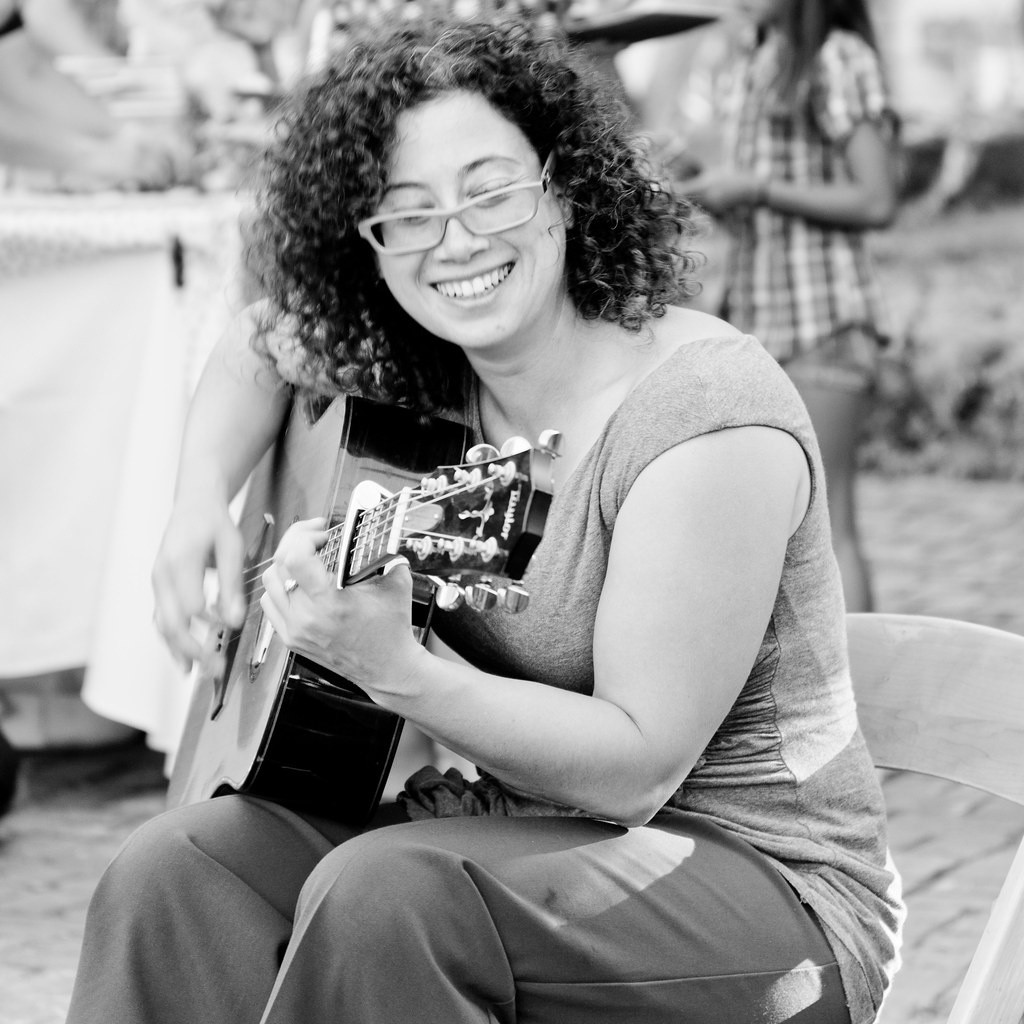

[359,150,557,255]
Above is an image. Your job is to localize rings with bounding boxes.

[283,578,301,594]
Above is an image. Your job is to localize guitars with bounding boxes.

[164,380,562,821]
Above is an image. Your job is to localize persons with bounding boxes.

[651,0,900,616]
[65,19,908,1024]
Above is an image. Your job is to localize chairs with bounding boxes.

[840,607,1024,1024]
